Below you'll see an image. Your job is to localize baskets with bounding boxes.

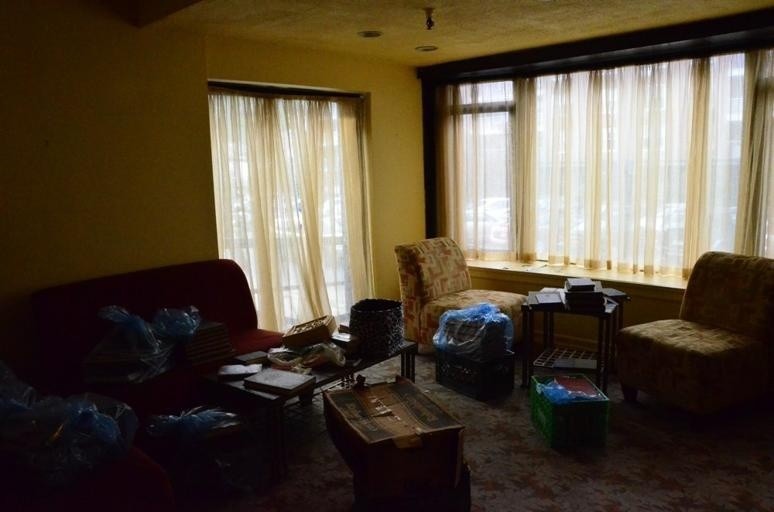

[350,299,405,357]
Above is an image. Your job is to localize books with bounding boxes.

[527,277,628,313]
[218,364,263,379]
[235,350,272,367]
[244,368,317,397]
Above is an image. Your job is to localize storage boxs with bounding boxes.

[320,375,471,510]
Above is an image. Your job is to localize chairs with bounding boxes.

[393,236,527,374]
[621,253,774,419]
[4,392,204,511]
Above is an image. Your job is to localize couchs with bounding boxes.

[13,257,282,443]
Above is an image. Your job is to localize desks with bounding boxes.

[519,289,627,397]
